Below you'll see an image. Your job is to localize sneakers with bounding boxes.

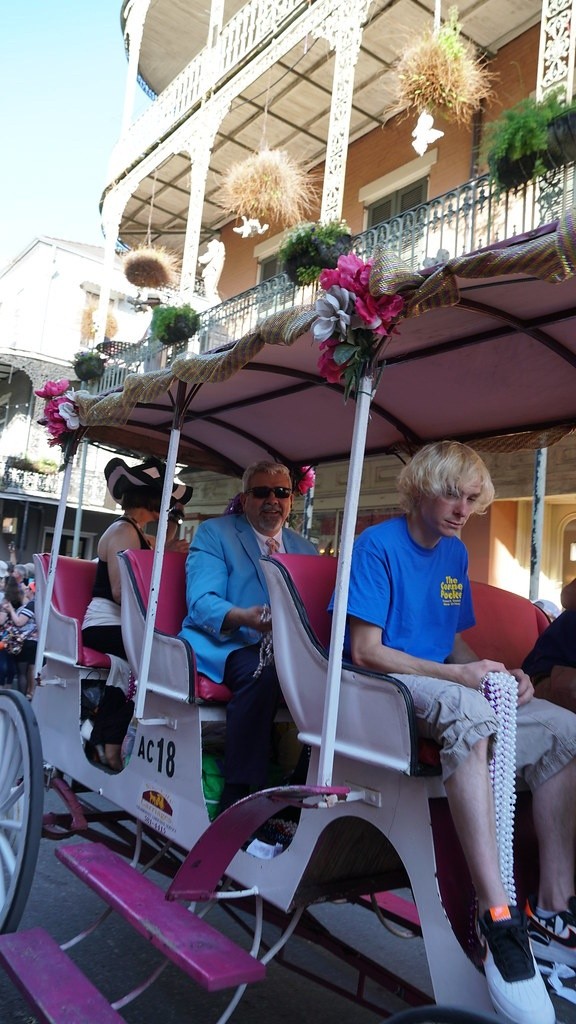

[474,902,556,1024]
[523,896,576,969]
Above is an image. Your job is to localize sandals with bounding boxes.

[26,692,33,700]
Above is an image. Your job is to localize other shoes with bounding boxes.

[105,744,122,772]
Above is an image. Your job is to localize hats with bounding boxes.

[104,458,193,506]
[0,561,10,578]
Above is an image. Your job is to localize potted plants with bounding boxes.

[213,149,325,224]
[123,243,182,289]
[376,6,503,131]
[151,303,199,345]
[478,85,576,204]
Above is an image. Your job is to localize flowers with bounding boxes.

[288,466,316,530]
[71,352,100,366]
[310,251,407,406]
[35,379,80,473]
[277,219,352,287]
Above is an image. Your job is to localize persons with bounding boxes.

[521,574,576,715]
[330,439,576,1024]
[174,460,318,798]
[534,599,559,623]
[0,541,45,702]
[79,458,191,774]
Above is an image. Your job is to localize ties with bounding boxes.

[266,538,280,555]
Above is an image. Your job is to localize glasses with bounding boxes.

[245,486,292,498]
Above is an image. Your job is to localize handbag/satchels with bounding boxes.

[0,605,38,655]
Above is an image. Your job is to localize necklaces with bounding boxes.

[254,604,275,680]
[123,513,154,550]
[477,670,520,908]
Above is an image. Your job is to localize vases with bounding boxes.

[285,235,352,286]
[74,357,106,380]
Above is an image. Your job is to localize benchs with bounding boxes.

[116,548,287,707]
[33,554,111,671]
[260,554,552,776]
